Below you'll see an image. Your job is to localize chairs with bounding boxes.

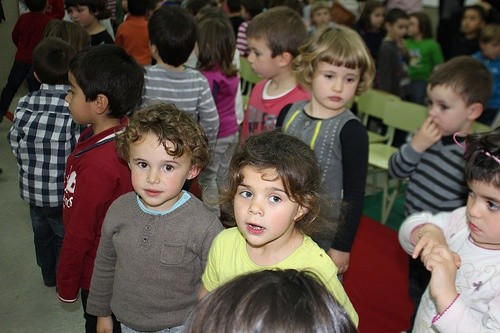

[240,57,492,226]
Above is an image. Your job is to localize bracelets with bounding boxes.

[417,223,425,241]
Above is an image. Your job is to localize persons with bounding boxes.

[235,0,500,127]
[197,129,360,332]
[183,266,359,333]
[197,16,237,218]
[142,3,221,181]
[85,101,228,333]
[397,135,500,333]
[387,55,493,333]
[240,5,309,146]
[0,0,152,124]
[8,36,89,288]
[273,22,377,284]
[55,44,146,320]
